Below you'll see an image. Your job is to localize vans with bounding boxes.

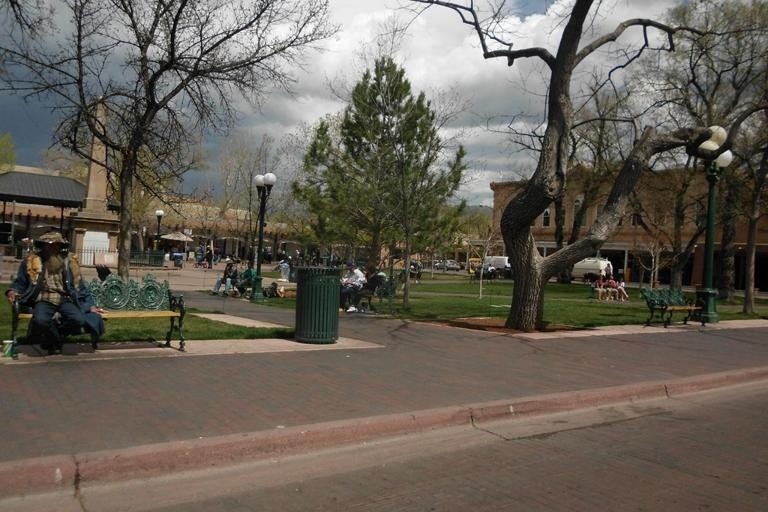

[572,256,613,282]
[480,255,512,277]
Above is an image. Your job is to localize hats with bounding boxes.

[227,260,235,263]
[347,262,353,269]
[33,232,72,249]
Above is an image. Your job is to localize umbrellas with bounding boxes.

[160,232,194,242]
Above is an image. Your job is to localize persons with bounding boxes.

[186,244,303,298]
[596,263,629,302]
[347,266,377,313]
[488,264,496,279]
[5,231,108,355]
[339,263,365,312]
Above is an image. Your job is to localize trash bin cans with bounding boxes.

[294,266,344,344]
[444,266,448,271]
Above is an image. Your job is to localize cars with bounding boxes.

[435,259,461,272]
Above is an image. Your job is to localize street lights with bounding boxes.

[249,172,277,302]
[691,125,733,324]
[155,210,164,251]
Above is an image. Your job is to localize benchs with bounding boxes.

[344,275,402,316]
[639,286,708,329]
[9,273,186,360]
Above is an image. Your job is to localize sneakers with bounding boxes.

[339,307,358,313]
[209,292,228,297]
